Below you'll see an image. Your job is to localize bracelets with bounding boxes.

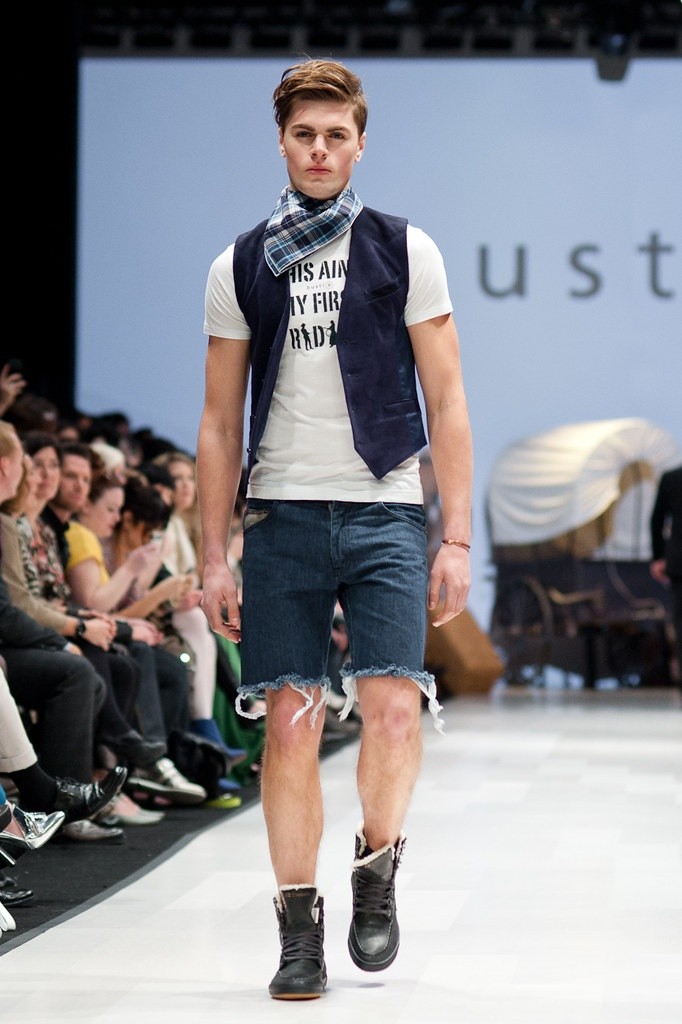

[442,539,470,551]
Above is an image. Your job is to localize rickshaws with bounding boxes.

[485,415,669,694]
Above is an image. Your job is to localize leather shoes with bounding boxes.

[64,819,126,842]
[0,871,36,908]
[19,767,127,825]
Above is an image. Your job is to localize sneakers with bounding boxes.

[185,713,248,790]
[129,758,207,801]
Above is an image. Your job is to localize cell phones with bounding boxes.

[187,567,193,574]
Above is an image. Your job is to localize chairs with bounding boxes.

[539,558,666,641]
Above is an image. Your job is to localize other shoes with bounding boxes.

[99,806,165,826]
[207,793,242,808]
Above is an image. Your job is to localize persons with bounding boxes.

[195,61,471,999]
[651,465,682,692]
[0,366,359,932]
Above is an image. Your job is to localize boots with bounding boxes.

[269,884,326,999]
[347,830,405,971]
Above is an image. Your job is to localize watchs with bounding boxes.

[77,618,86,637]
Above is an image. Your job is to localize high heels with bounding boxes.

[0,804,65,866]
[113,730,167,769]
